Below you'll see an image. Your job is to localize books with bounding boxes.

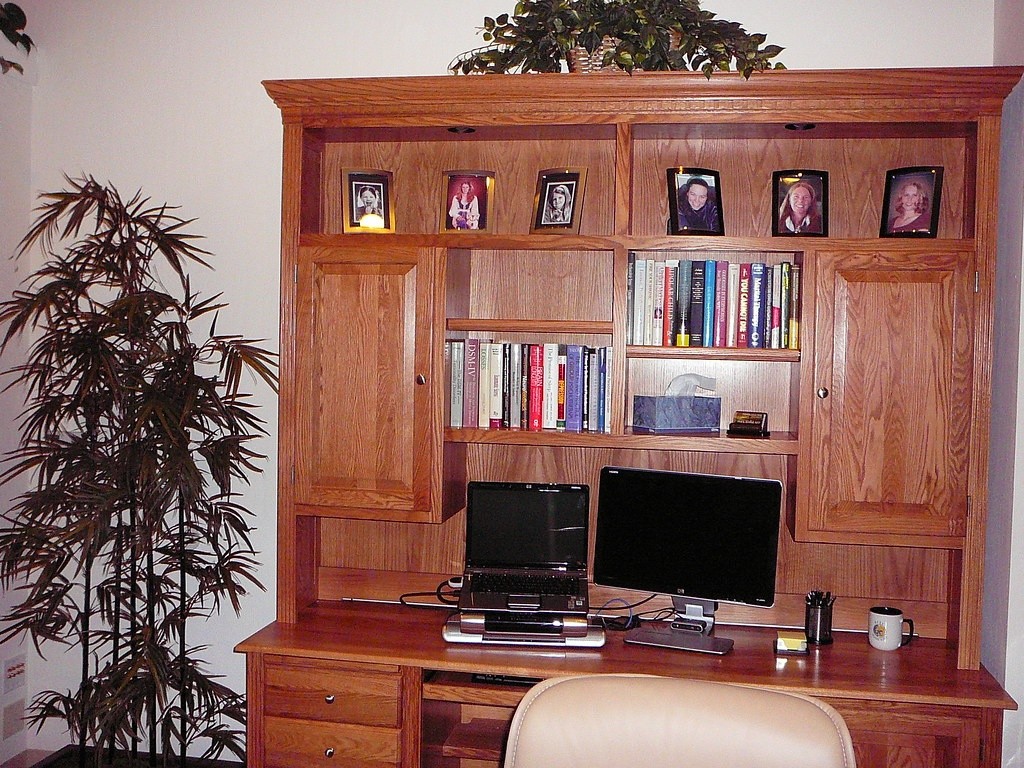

[450,337,612,434]
[626,254,802,351]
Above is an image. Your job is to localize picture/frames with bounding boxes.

[878,164,944,238]
[666,167,724,236]
[340,166,399,235]
[772,169,830,237]
[439,170,496,233]
[530,166,588,236]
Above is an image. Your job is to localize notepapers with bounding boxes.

[777,631,806,651]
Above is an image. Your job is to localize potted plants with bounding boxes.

[445,0,787,83]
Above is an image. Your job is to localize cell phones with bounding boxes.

[773,639,810,656]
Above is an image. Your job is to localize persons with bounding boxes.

[541,186,571,225]
[449,183,480,230]
[886,181,930,233]
[680,179,719,230]
[354,183,381,219]
[777,184,821,233]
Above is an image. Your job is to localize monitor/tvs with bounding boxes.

[594,466,782,655]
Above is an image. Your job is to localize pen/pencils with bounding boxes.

[804,589,837,609]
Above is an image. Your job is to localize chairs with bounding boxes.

[500,676,856,768]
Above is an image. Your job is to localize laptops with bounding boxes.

[458,481,589,616]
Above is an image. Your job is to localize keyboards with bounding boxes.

[470,673,543,686]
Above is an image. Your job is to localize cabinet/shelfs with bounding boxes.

[232,63,1024,768]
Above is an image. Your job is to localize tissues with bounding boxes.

[633,373,721,434]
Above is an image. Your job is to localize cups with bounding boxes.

[868,607,914,651]
[805,603,833,644]
